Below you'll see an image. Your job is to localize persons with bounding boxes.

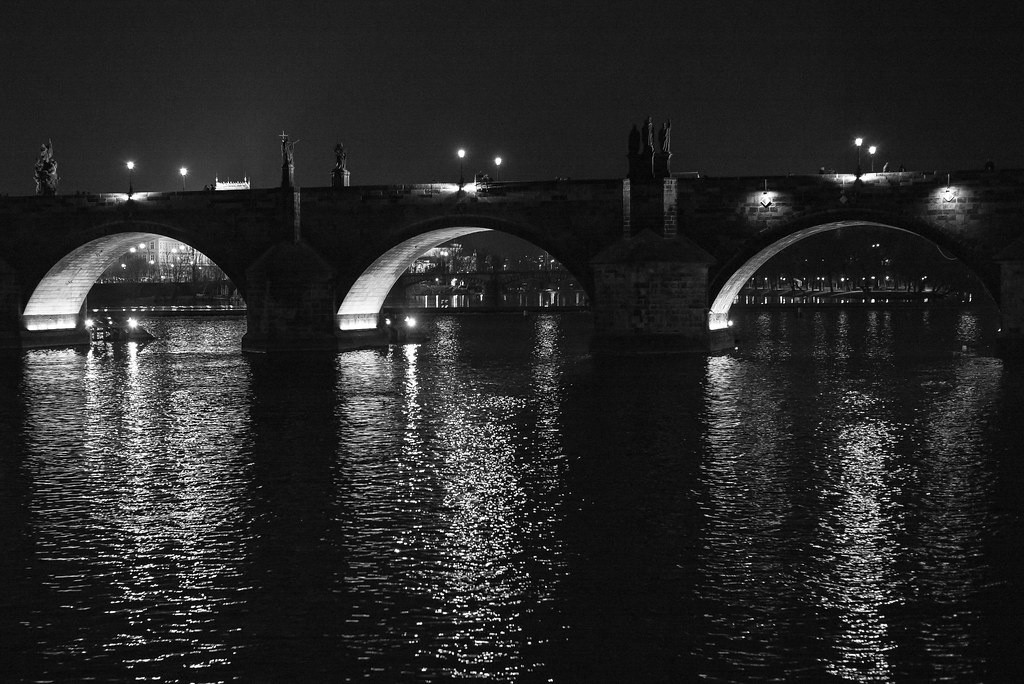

[984,157,994,171]
[881,161,907,173]
[818,165,838,174]
[38,139,54,163]
[628,115,672,154]
[283,136,300,166]
[204,184,215,191]
[334,142,347,169]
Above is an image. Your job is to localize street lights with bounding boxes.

[495,157,501,181]
[127,162,134,192]
[855,137,862,173]
[869,146,876,173]
[458,149,465,183]
[180,168,187,191]
